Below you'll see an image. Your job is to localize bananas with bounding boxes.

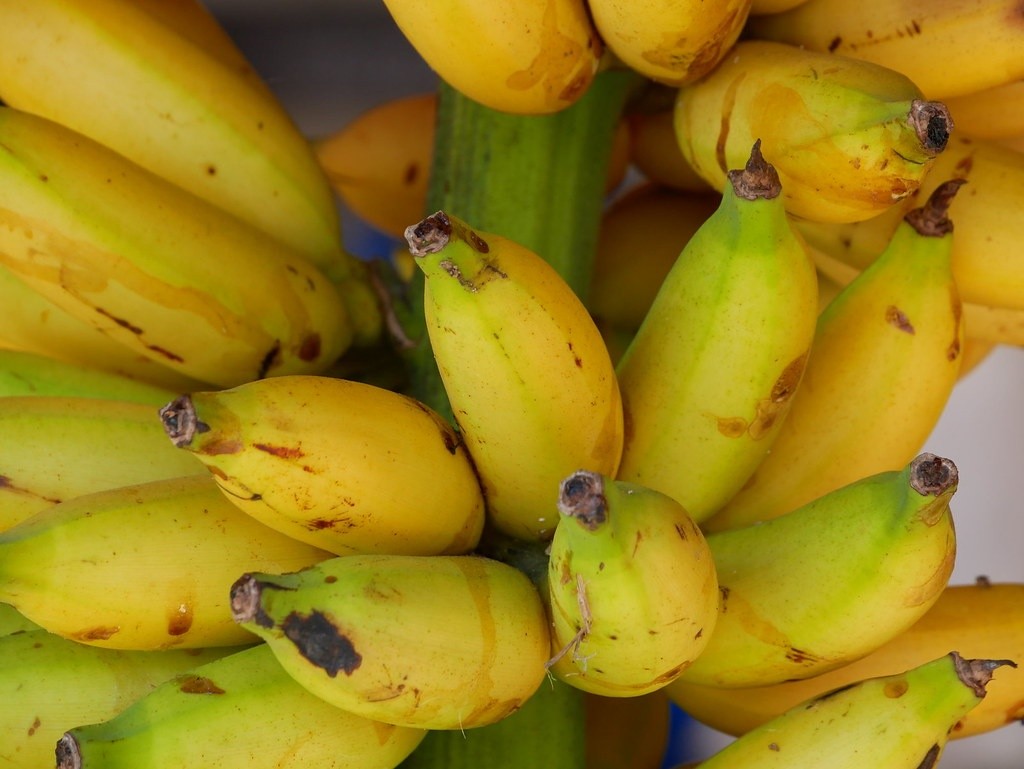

[0,3,1024,769]
